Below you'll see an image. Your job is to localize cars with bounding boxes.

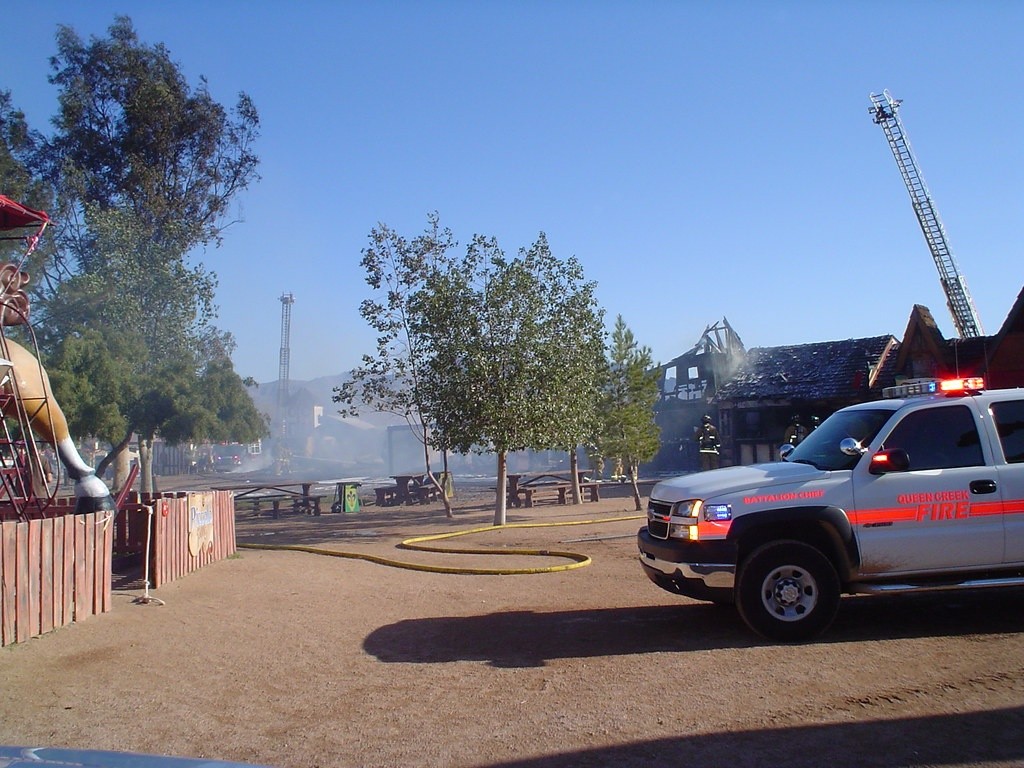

[638,377,1024,646]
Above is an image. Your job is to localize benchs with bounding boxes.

[233,493,326,522]
[374,481,438,508]
[487,480,606,509]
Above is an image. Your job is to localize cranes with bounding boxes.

[868,88,987,339]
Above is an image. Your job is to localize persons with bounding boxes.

[13,440,54,498]
[694,414,722,471]
[589,454,639,481]
[783,414,822,446]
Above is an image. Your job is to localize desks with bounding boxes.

[210,481,319,520]
[389,473,428,507]
[506,469,593,508]
